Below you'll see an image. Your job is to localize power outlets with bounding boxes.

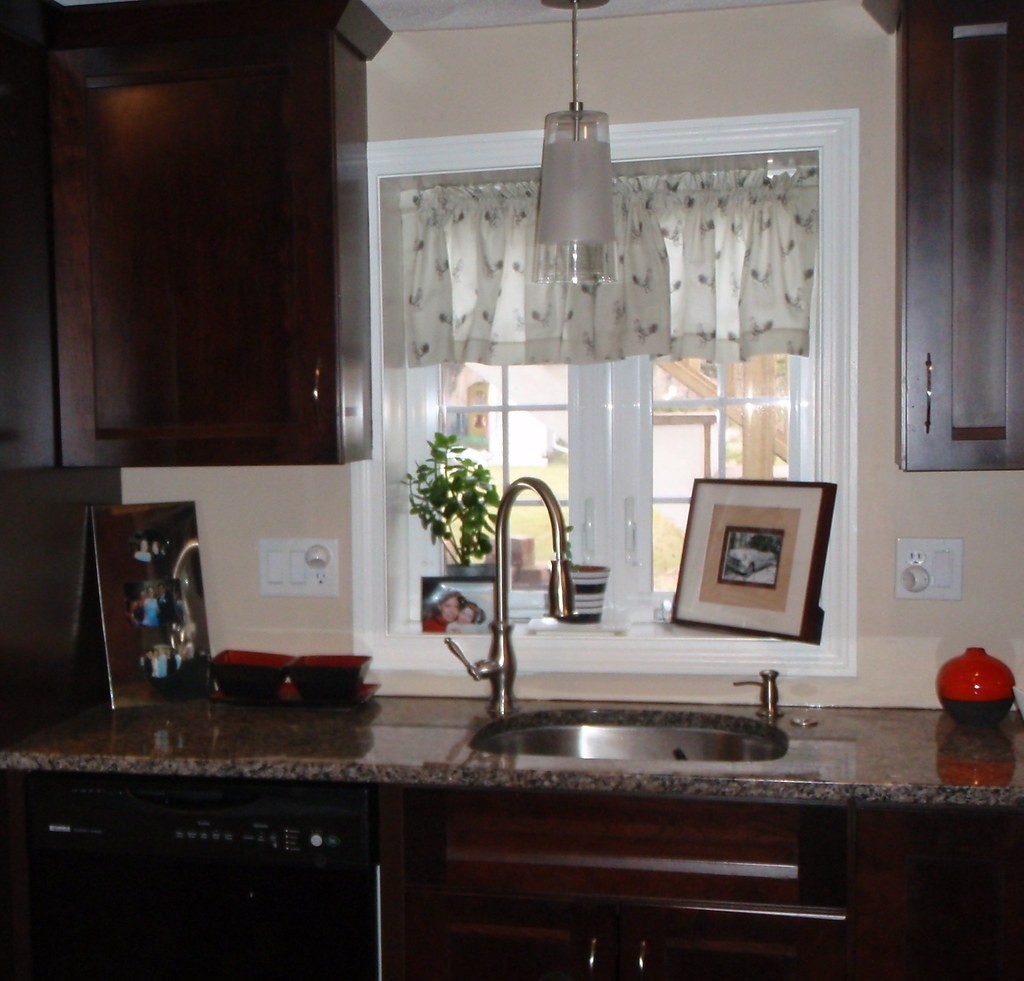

[258,537,340,597]
[893,537,963,600]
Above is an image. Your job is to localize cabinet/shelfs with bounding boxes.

[60,0,393,481]
[375,785,1024,981]
[896,0,1024,472]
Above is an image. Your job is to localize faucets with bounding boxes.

[491,474,574,716]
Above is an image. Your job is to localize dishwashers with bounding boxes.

[27,778,383,981]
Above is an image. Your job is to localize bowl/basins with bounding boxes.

[212,649,294,700]
[287,655,371,701]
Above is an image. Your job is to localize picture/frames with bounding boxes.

[88,500,211,710]
[669,479,837,645]
[420,576,499,637]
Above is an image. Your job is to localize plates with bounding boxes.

[205,682,290,706]
[284,682,380,705]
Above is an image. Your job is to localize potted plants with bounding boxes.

[552,527,610,624]
[402,433,500,578]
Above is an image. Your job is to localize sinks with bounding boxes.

[465,702,791,769]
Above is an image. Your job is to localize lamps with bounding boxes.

[531,0,618,286]
